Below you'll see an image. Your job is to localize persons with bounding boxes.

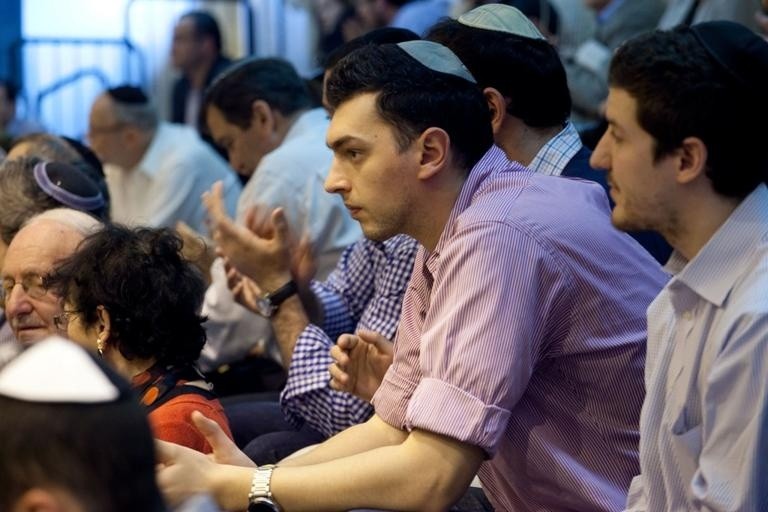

[1,0,768,511]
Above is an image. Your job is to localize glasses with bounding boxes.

[0,274,61,301]
[53,309,99,331]
